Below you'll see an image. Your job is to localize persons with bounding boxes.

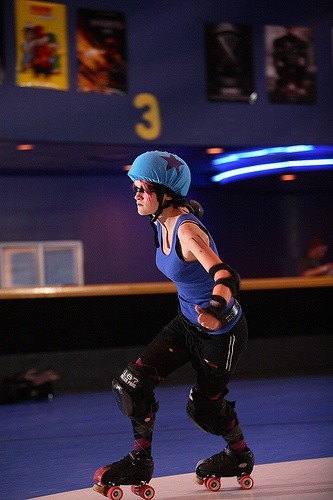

[92,149,255,485]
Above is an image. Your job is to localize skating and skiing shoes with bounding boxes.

[194,447,254,493]
[91,450,154,500]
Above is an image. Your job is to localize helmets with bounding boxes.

[127,150,191,198]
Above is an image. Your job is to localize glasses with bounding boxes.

[134,187,156,193]
